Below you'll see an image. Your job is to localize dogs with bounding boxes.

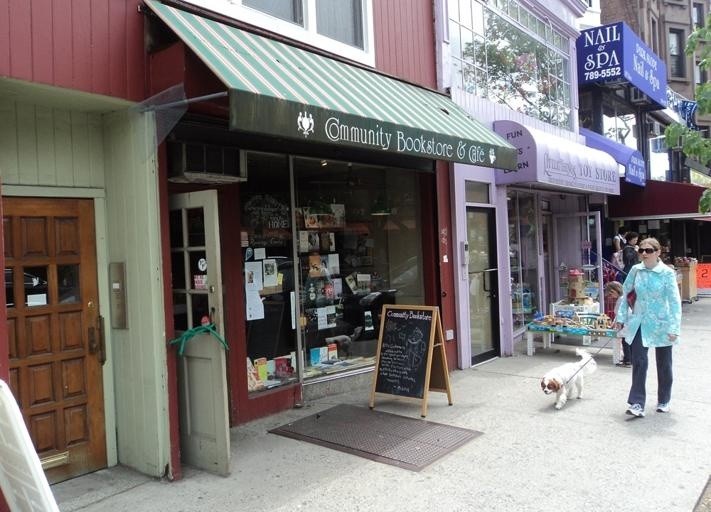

[540,347,597,410]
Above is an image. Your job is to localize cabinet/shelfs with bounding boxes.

[298,226,374,308]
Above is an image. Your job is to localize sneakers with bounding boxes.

[627,404,645,418]
[657,404,668,412]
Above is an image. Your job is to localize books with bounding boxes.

[310,344,337,364]
[254,351,305,380]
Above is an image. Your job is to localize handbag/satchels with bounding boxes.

[627,292,637,310]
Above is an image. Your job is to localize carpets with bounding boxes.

[267,404,486,474]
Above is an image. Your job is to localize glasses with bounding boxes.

[637,247,657,254]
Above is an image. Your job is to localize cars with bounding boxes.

[5,268,48,307]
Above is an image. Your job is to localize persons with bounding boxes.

[607,281,632,368]
[615,237,682,417]
[613,226,639,284]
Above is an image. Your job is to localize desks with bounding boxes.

[548,301,600,343]
[525,320,626,368]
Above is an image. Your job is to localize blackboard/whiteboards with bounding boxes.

[370,304,438,401]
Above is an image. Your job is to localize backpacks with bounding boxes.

[612,250,625,270]
